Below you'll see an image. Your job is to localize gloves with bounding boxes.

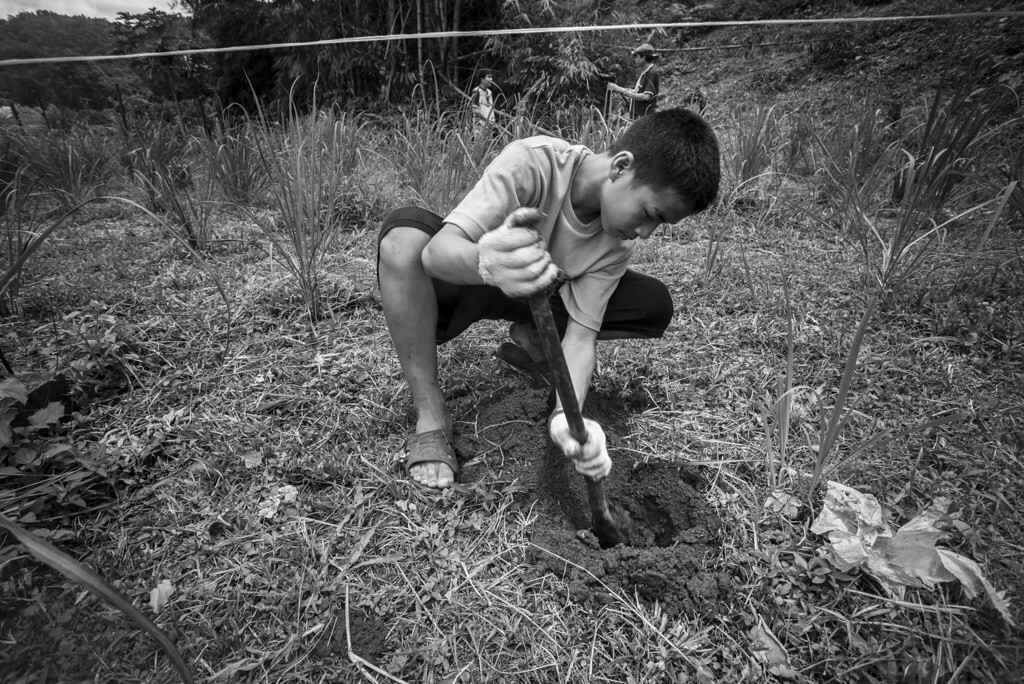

[546,405,614,484]
[476,206,560,298]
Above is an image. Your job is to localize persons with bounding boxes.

[376,109,721,490]
[470,68,496,137]
[610,44,659,116]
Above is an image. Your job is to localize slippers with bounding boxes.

[496,342,554,388]
[402,429,459,489]
